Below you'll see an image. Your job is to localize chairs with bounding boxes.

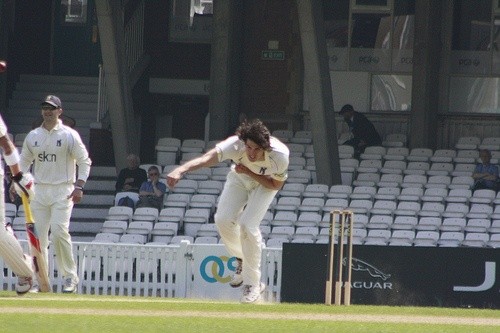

[3,128,500,276]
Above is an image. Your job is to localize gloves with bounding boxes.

[10,171,37,200]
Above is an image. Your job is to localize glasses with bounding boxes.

[41,106,61,111]
[149,172,159,176]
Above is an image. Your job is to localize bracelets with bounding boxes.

[75,185,85,191]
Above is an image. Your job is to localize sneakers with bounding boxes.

[229,257,243,287]
[29,283,42,294]
[15,254,35,294]
[61,277,80,294]
[240,282,266,303]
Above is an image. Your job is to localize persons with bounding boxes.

[115,153,148,193]
[0,113,34,296]
[138,166,166,214]
[167,118,290,304]
[17,94,93,293]
[340,103,383,159]
[472,148,500,192]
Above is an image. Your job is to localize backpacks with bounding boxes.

[118,196,134,214]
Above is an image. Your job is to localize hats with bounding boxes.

[40,94,62,109]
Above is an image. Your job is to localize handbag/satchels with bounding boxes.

[137,195,163,210]
